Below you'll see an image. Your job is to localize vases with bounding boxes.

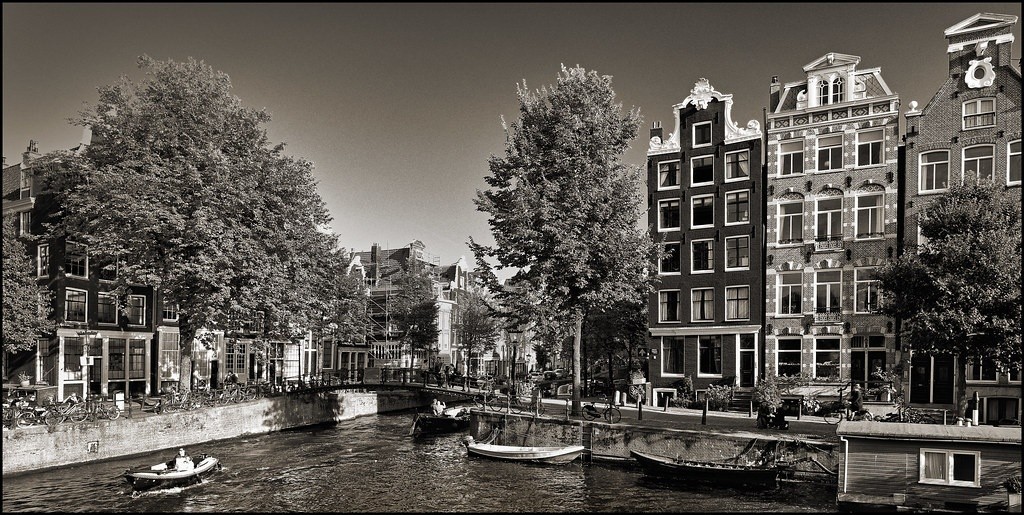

[20,381,30,387]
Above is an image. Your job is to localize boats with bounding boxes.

[462,436,584,465]
[412,406,479,433]
[629,450,790,485]
[123,449,221,492]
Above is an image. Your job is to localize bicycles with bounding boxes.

[509,394,545,416]
[474,393,502,412]
[824,402,872,425]
[582,401,621,423]
[16,400,120,428]
[153,384,256,414]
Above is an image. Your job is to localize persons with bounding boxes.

[435,362,456,389]
[509,387,517,404]
[2,395,39,424]
[65,393,78,420]
[173,448,194,466]
[343,364,349,384]
[42,395,55,423]
[358,365,364,381]
[223,370,238,400]
[539,374,549,393]
[422,360,428,386]
[431,399,446,417]
[846,384,863,421]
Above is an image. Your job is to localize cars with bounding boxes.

[543,371,556,380]
[526,372,544,382]
[555,369,568,379]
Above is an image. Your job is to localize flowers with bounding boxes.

[18,371,32,383]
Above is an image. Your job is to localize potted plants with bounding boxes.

[632,369,646,384]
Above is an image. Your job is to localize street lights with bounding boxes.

[295,337,305,390]
[508,330,523,400]
